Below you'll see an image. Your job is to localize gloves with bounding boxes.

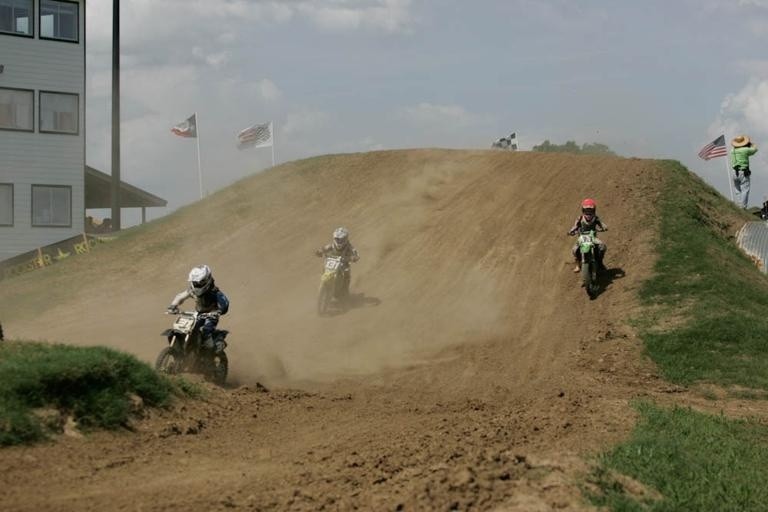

[167,304,178,315]
[316,249,359,263]
[569,227,608,236]
[209,311,218,320]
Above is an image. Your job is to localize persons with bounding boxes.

[313,227,361,298]
[566,199,609,273]
[167,264,230,383]
[729,134,758,210]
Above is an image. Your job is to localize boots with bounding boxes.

[573,257,582,273]
[597,258,603,270]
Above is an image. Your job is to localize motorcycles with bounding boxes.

[566,228,609,300]
[311,248,360,318]
[156,307,231,384]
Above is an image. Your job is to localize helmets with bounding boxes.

[187,264,214,298]
[332,227,350,249]
[581,198,596,222]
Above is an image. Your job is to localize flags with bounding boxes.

[168,113,198,139]
[237,121,274,149]
[489,131,518,152]
[698,135,727,162]
